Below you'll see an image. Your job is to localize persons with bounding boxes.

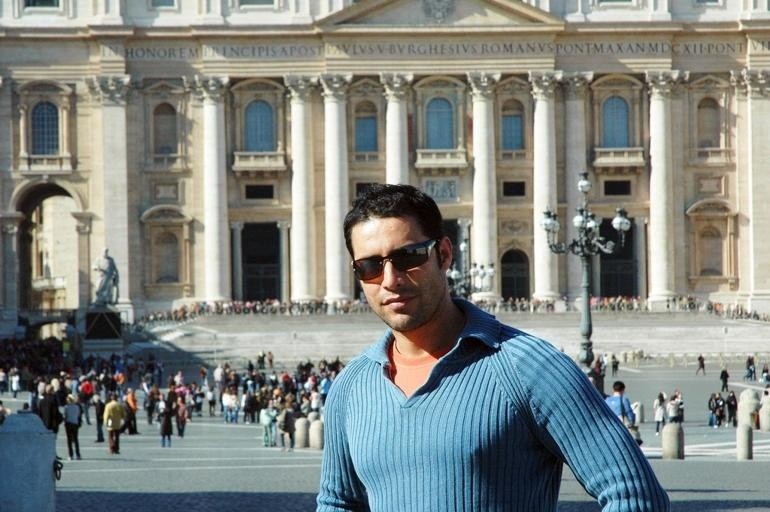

[666,295,770,322]
[121,297,373,344]
[561,348,685,435]
[92,247,118,305]
[474,295,569,313]
[695,354,770,430]
[589,295,648,312]
[312,184,672,512]
[0,333,345,461]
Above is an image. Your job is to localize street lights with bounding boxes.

[446,237,498,299]
[543,172,631,376]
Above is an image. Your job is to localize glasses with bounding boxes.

[349,236,437,283]
